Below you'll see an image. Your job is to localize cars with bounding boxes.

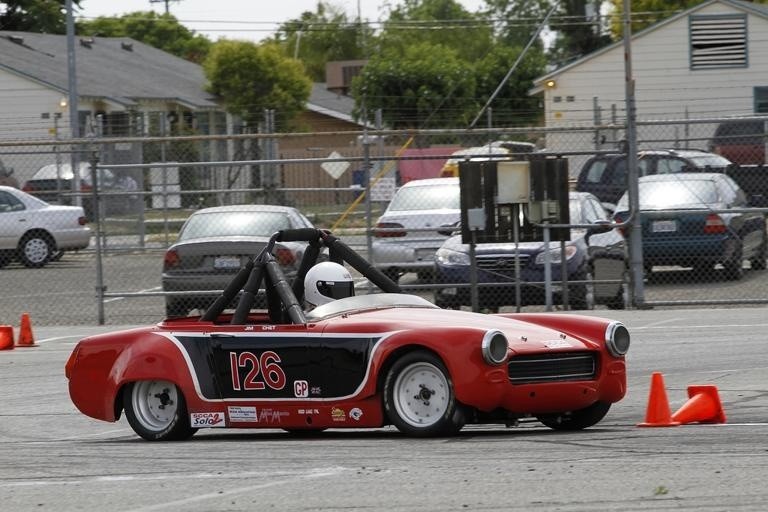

[0,162,129,268]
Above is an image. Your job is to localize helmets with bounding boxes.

[303,260,356,307]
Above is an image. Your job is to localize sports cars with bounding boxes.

[65,228,630,441]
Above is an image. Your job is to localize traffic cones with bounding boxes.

[0,313,40,350]
[635,371,727,428]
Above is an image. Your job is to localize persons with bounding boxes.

[304,261,355,322]
